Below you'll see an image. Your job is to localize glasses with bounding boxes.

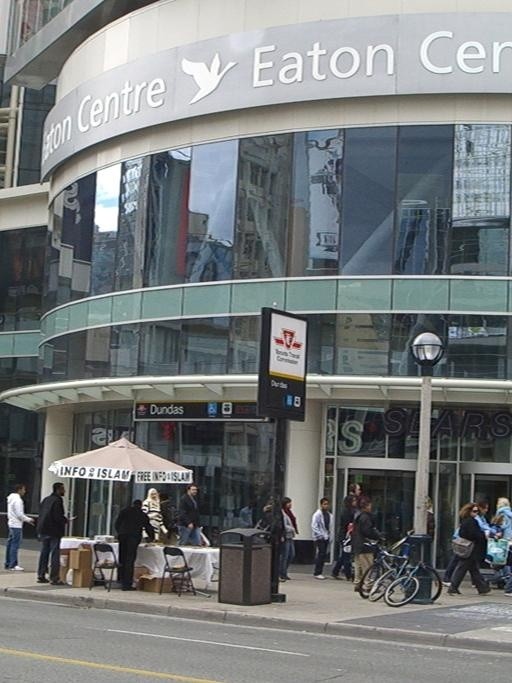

[471,509,480,513]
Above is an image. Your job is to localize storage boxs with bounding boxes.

[141,575,170,593]
[70,549,92,569]
[73,570,93,587]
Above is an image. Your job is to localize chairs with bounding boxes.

[89,544,120,592]
[159,547,196,597]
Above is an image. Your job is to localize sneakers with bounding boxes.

[278,577,285,582]
[50,580,66,585]
[479,589,494,596]
[504,591,511,598]
[440,580,451,587]
[448,590,463,596]
[36,577,50,583]
[9,565,24,572]
[313,574,325,580]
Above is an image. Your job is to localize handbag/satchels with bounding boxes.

[487,538,509,566]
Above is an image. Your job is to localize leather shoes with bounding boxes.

[122,586,136,590]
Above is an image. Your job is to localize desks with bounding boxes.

[59,537,220,593]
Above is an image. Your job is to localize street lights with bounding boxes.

[411,333,447,600]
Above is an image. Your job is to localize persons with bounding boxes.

[178,484,203,546]
[280,497,299,579]
[448,502,492,596]
[114,500,155,591]
[354,496,382,593]
[260,497,285,583]
[331,495,357,582]
[142,488,168,544]
[349,483,361,497]
[239,497,257,531]
[441,503,502,588]
[491,497,512,542]
[5,482,34,571]
[37,482,67,586]
[311,498,336,580]
[157,492,178,544]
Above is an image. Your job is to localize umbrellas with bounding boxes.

[47,437,194,488]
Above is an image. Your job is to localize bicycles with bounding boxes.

[369,555,425,607]
[359,531,443,604]
